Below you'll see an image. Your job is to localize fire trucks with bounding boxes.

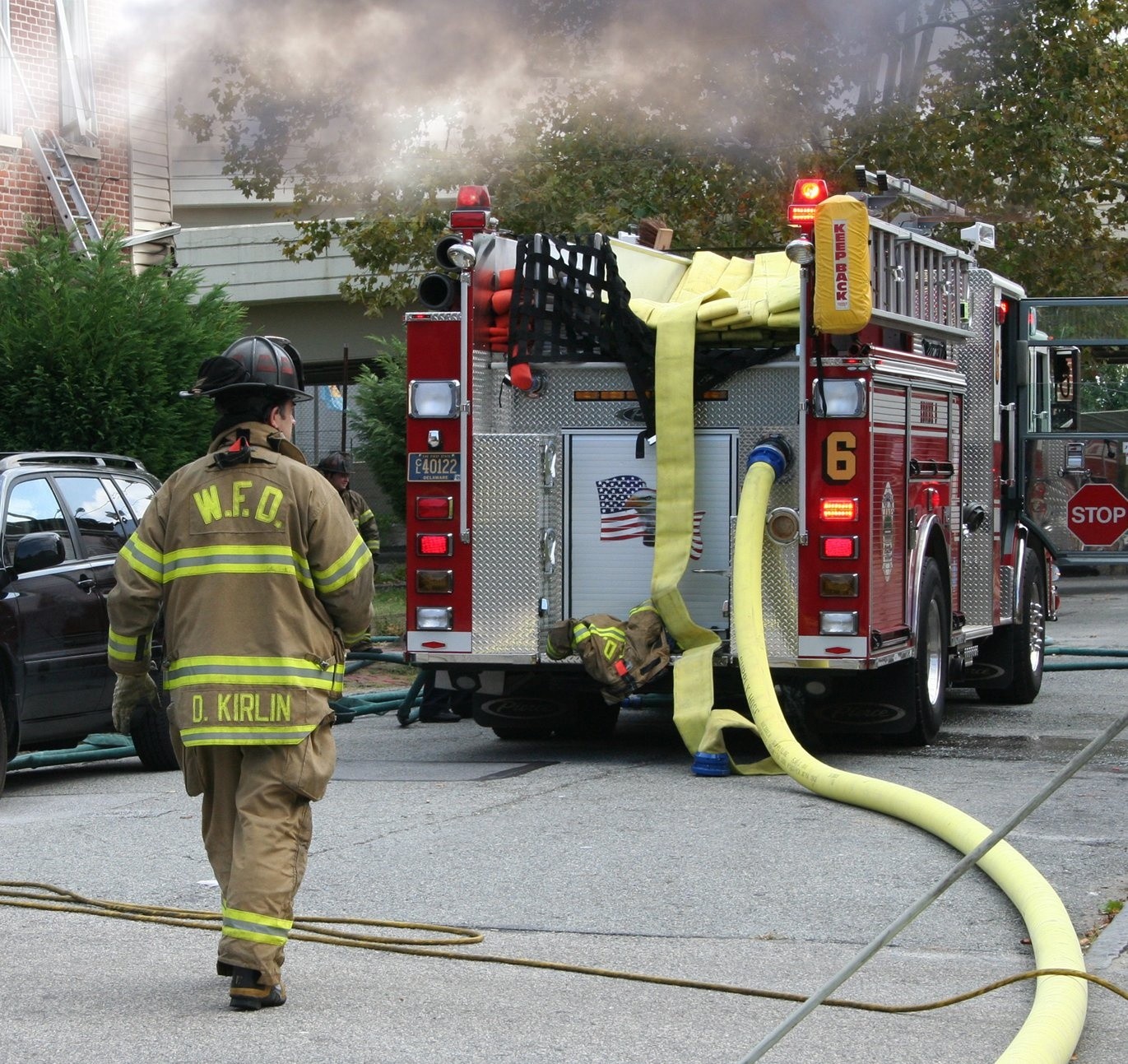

[406,164,1128,746]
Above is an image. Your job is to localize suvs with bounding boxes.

[0,451,179,793]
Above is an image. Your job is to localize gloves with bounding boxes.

[112,672,164,735]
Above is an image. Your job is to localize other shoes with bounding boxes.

[217,958,264,977]
[230,976,287,1011]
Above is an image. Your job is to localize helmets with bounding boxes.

[316,452,358,474]
[198,336,313,403]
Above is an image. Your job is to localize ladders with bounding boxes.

[20,123,103,261]
[867,196,975,345]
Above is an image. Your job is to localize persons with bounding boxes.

[317,451,383,652]
[106,333,374,1011]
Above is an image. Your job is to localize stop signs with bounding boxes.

[1067,484,1128,546]
